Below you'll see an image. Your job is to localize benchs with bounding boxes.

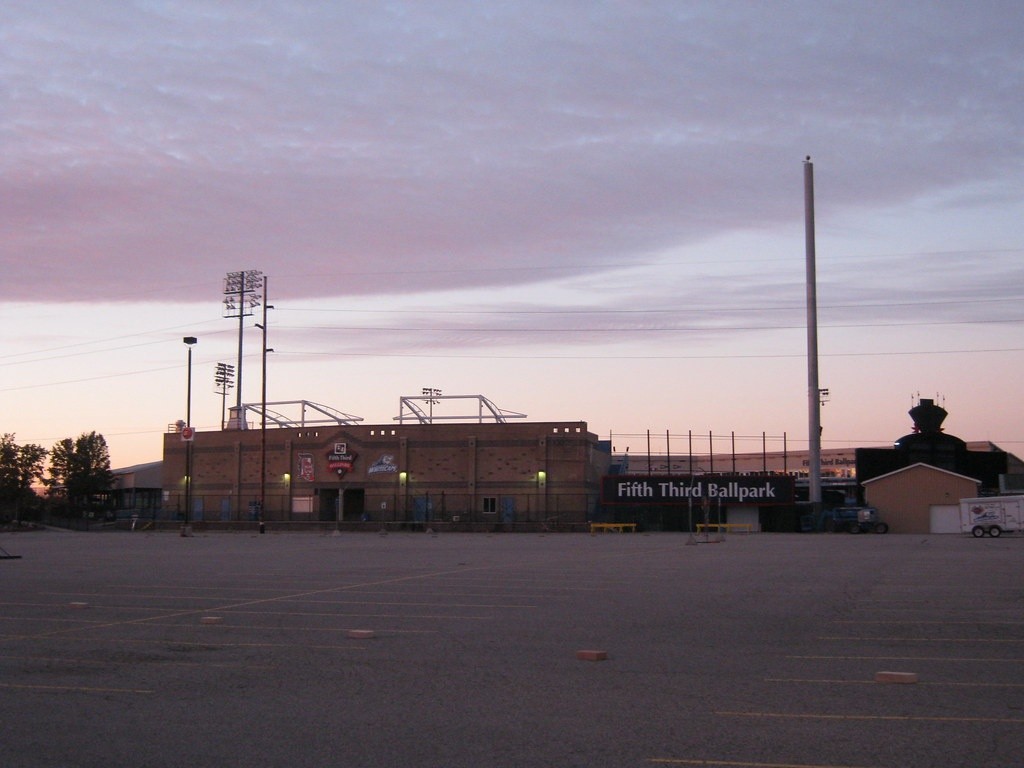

[696,524,751,535]
[591,523,636,535]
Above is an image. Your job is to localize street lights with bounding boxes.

[184,336,197,524]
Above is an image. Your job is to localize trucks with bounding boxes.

[959,495,1024,538]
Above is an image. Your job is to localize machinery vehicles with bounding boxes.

[801,505,889,534]
